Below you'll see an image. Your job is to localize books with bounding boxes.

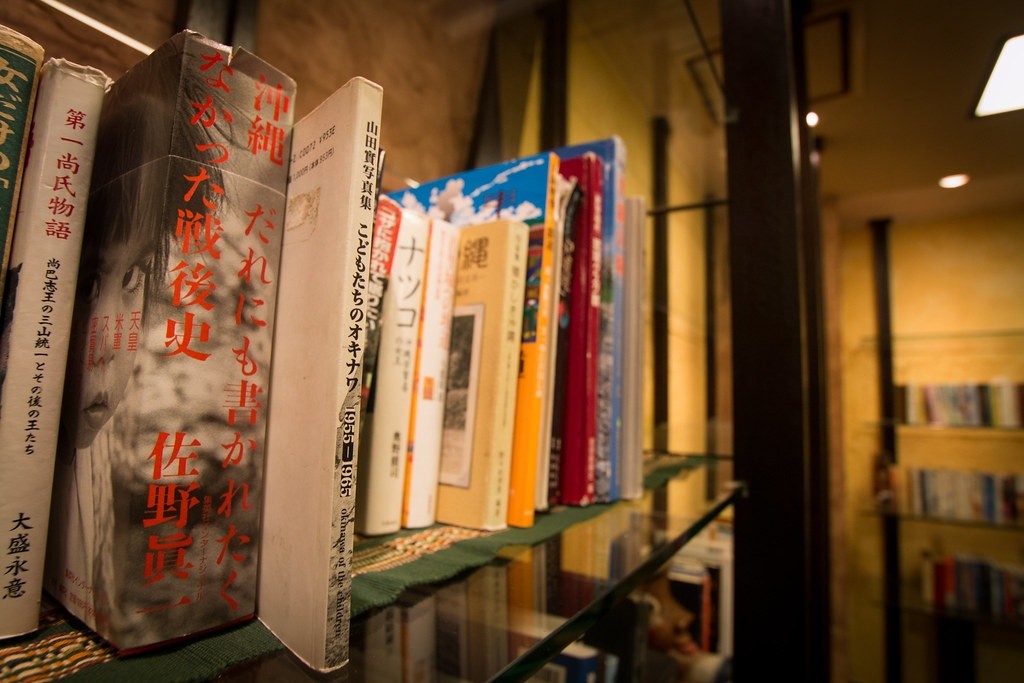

[887,463,1024,525]
[892,376,1023,431]
[249,456,736,682]
[908,547,1023,625]
[0,18,652,676]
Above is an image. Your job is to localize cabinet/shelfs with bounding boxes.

[872,328,1024,683]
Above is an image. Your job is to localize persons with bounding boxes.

[608,563,701,682]
